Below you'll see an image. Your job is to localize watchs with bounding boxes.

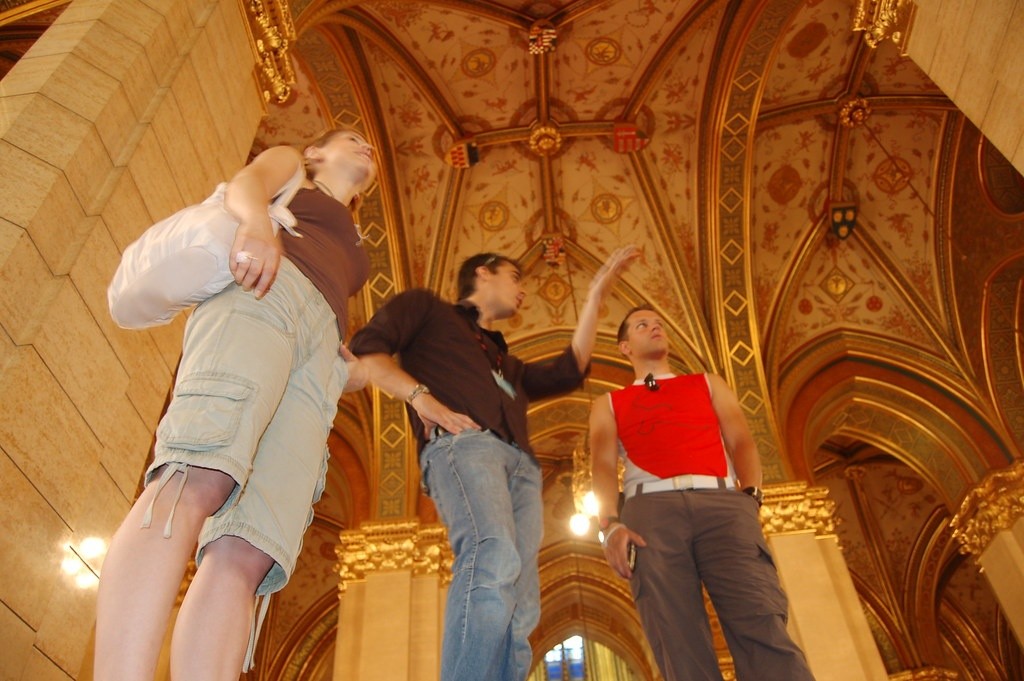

[598,517,620,532]
[743,487,763,507]
[407,384,430,404]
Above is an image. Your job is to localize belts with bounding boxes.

[623,476,736,500]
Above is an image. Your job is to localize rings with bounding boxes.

[236,252,256,263]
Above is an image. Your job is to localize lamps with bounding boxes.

[553,185,625,540]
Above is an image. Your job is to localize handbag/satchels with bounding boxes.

[107,166,307,330]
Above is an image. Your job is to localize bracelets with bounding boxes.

[602,525,626,547]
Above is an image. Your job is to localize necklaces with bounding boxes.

[313,179,334,199]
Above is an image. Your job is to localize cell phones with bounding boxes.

[626,543,635,571]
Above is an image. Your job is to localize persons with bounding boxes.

[348,243,644,681]
[589,306,816,681]
[94,128,378,681]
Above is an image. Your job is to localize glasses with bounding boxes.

[645,372,659,390]
